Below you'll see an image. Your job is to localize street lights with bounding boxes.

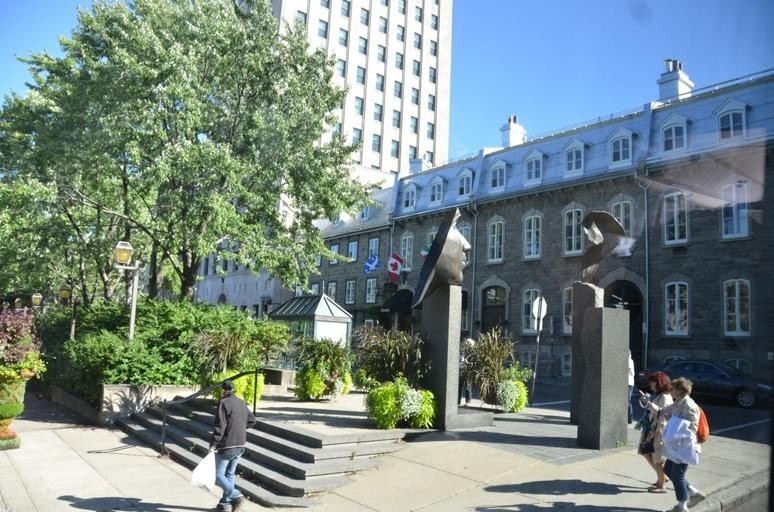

[110,238,141,342]
[59,283,78,341]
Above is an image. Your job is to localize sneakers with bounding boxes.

[687,491,705,507]
[648,486,666,492]
[652,478,669,486]
[666,505,687,512]
[216,503,232,512]
[231,497,249,512]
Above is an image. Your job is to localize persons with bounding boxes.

[457,344,474,405]
[209,381,257,510]
[644,376,707,509]
[626,352,638,424]
[638,371,675,495]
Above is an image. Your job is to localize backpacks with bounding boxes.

[696,407,708,442]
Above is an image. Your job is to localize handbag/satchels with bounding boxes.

[640,442,655,453]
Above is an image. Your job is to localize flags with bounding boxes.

[362,250,382,275]
[388,251,404,282]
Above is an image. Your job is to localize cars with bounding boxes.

[637,359,773,410]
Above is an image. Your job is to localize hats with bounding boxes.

[222,380,236,390]
